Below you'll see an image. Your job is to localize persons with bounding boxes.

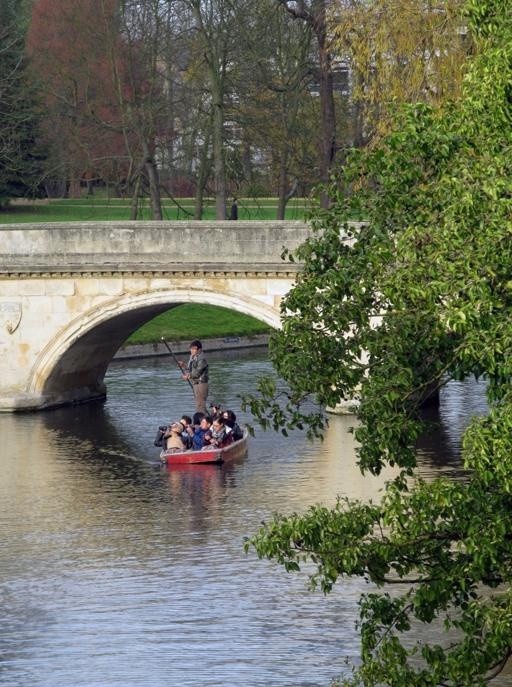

[177,340,209,415]
[230,197,239,219]
[155,401,242,452]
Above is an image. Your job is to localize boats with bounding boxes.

[159,421,251,467]
